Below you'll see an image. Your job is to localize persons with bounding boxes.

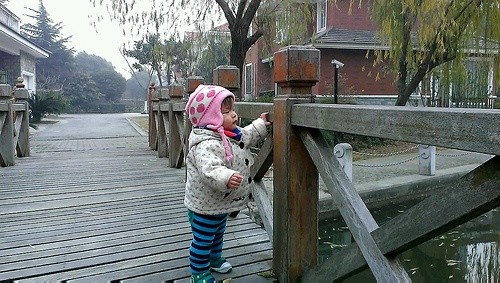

[184,84,272,283]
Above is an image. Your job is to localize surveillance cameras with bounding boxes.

[331,59,344,70]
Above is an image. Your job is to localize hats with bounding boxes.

[185,84,234,162]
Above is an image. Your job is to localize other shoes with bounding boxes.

[211,258,232,273]
[190,271,217,282]
